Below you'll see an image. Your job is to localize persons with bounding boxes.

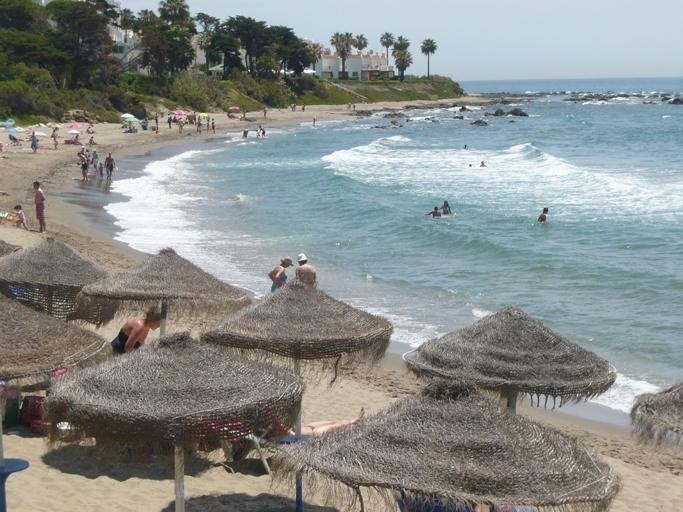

[8,134,23,143]
[468,163,472,167]
[266,254,292,294]
[30,124,96,151]
[479,161,485,168]
[439,201,452,215]
[424,206,442,218]
[312,117,316,128]
[228,109,247,121]
[243,125,266,139]
[9,201,31,232]
[77,146,115,181]
[537,205,549,225]
[264,108,267,118]
[142,112,215,131]
[290,103,305,112]
[31,182,51,235]
[463,144,467,150]
[122,122,138,133]
[269,407,368,438]
[107,305,163,354]
[293,251,317,288]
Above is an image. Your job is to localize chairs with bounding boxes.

[214,424,312,477]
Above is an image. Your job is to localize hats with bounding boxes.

[280,256,294,267]
[297,252,308,262]
